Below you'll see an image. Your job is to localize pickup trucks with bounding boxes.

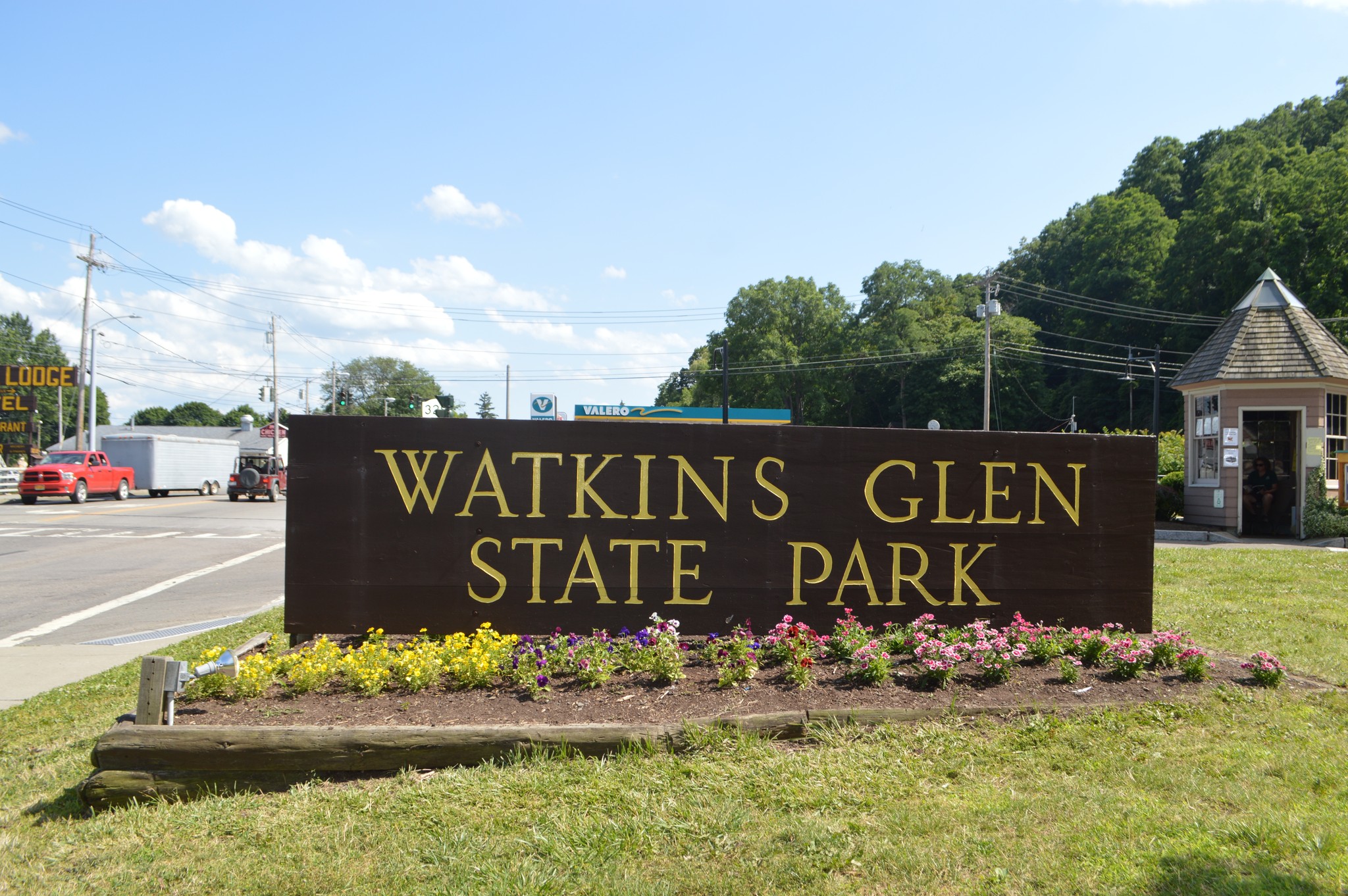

[17,451,136,504]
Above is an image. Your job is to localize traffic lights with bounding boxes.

[271,388,276,402]
[339,391,347,406]
[409,394,416,409]
[416,394,423,408]
[346,393,354,405]
[434,393,454,418]
[258,387,265,402]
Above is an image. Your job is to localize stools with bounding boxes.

[1250,502,1265,535]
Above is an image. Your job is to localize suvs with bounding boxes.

[226,455,287,503]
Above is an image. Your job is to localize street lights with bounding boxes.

[305,372,351,415]
[1117,344,1161,466]
[708,338,729,424]
[76,312,141,451]
